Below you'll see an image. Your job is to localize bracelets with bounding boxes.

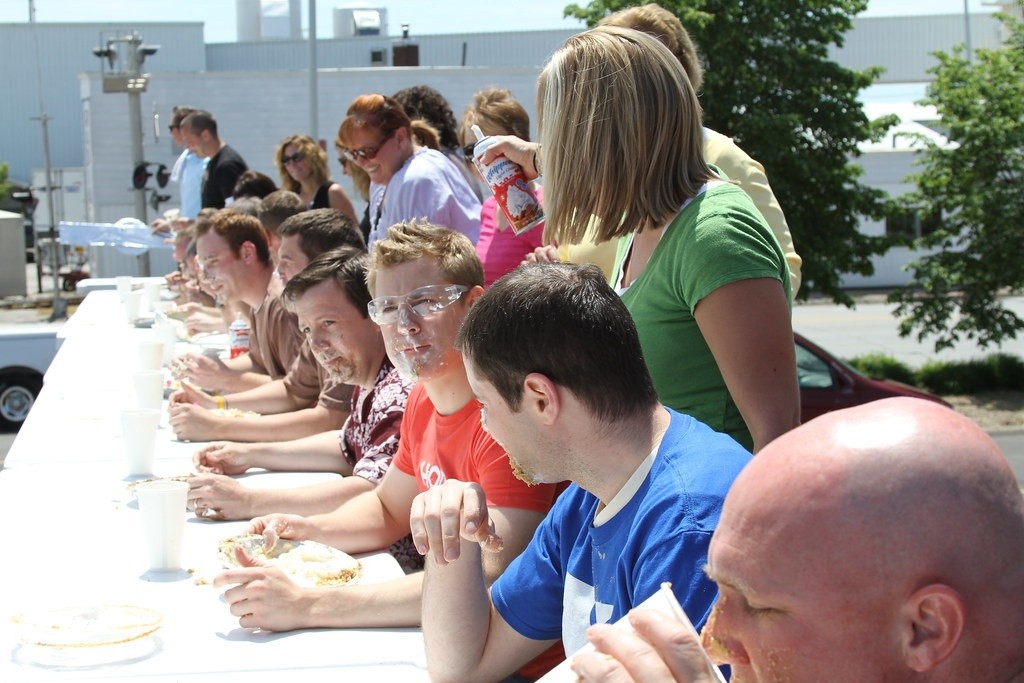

[216,396,224,410]
[225,398,228,409]
[533,146,541,176]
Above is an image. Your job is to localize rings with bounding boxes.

[193,499,197,509]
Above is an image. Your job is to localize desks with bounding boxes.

[0,275,429,683]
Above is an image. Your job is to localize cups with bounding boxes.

[165,209,180,219]
[120,409,162,470]
[124,294,144,324]
[144,278,162,304]
[137,481,191,574]
[533,581,727,683]
[134,366,163,409]
[113,276,130,301]
[144,337,165,366]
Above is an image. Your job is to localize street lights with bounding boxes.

[11,191,42,296]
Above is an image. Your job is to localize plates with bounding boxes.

[218,534,362,589]
[206,406,262,418]
[9,602,160,647]
[191,331,243,349]
[131,473,241,500]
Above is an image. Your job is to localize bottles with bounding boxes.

[471,125,546,235]
[229,311,252,358]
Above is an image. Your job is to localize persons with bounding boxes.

[185,245,426,576]
[481,3,802,304]
[332,86,484,253]
[212,218,566,683]
[568,396,1024,683]
[410,263,754,683]
[459,89,544,289]
[539,25,800,452]
[152,106,367,443]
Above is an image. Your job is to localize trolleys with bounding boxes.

[57,270,94,292]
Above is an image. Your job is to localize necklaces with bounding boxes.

[309,187,318,206]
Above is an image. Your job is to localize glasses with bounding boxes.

[344,134,392,162]
[168,124,179,132]
[369,283,476,326]
[463,144,475,157]
[281,152,307,165]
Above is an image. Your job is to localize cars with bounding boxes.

[791,331,955,428]
[0,324,69,432]
